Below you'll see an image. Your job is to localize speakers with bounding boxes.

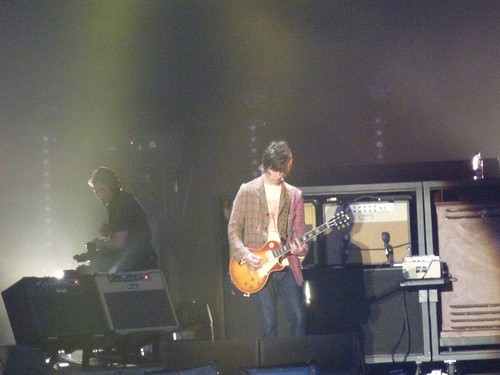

[322,195,418,266]
[296,199,323,270]
[92,269,179,331]
[1,276,110,344]
[156,334,366,375]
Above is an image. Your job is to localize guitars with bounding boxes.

[228,210,351,297]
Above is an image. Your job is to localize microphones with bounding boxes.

[382,232,390,260]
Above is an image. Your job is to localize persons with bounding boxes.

[227,141,308,337]
[88,167,152,274]
